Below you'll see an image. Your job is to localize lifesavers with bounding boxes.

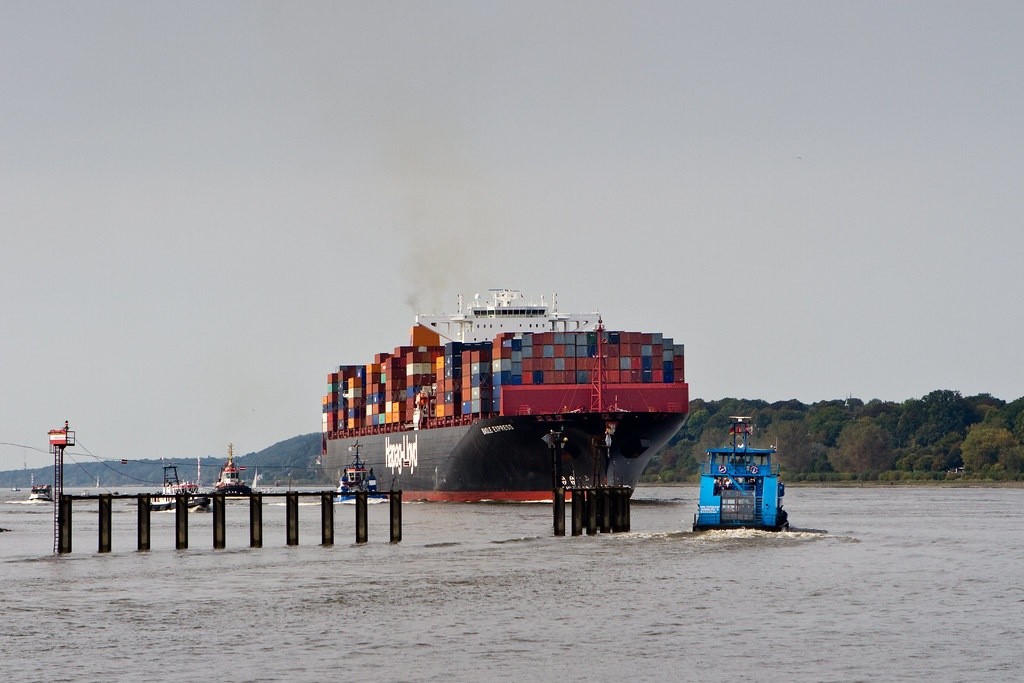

[718,464,727,474]
[750,466,759,474]
[601,414,609,420]
[616,413,623,420]
[536,415,564,421]
[719,478,730,489]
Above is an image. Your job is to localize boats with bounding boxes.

[329,437,390,504]
[691,417,790,537]
[28,483,54,502]
[11,486,21,491]
[144,456,212,512]
[208,440,255,501]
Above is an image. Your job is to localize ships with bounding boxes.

[317,287,693,506]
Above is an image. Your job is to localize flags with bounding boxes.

[729,422,754,435]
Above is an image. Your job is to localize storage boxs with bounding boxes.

[323,331,684,432]
